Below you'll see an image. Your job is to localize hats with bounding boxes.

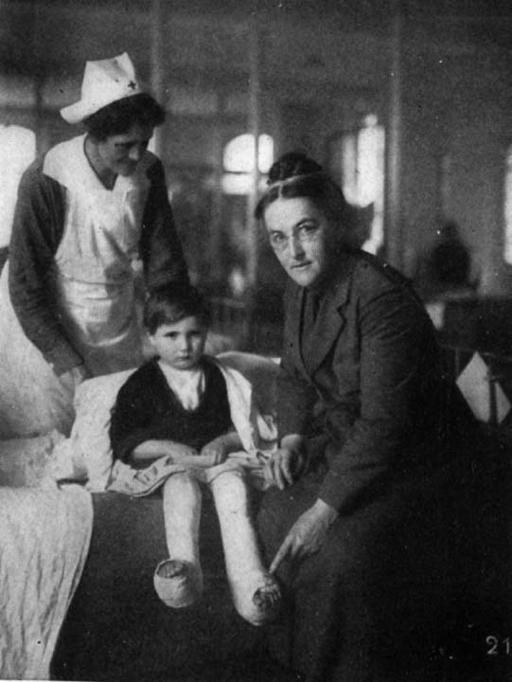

[58,52,145,125]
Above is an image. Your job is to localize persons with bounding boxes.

[418,236,480,299]
[257,148,484,682]
[0,50,192,488]
[111,278,282,626]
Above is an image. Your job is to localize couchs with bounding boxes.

[0,349,283,681]
